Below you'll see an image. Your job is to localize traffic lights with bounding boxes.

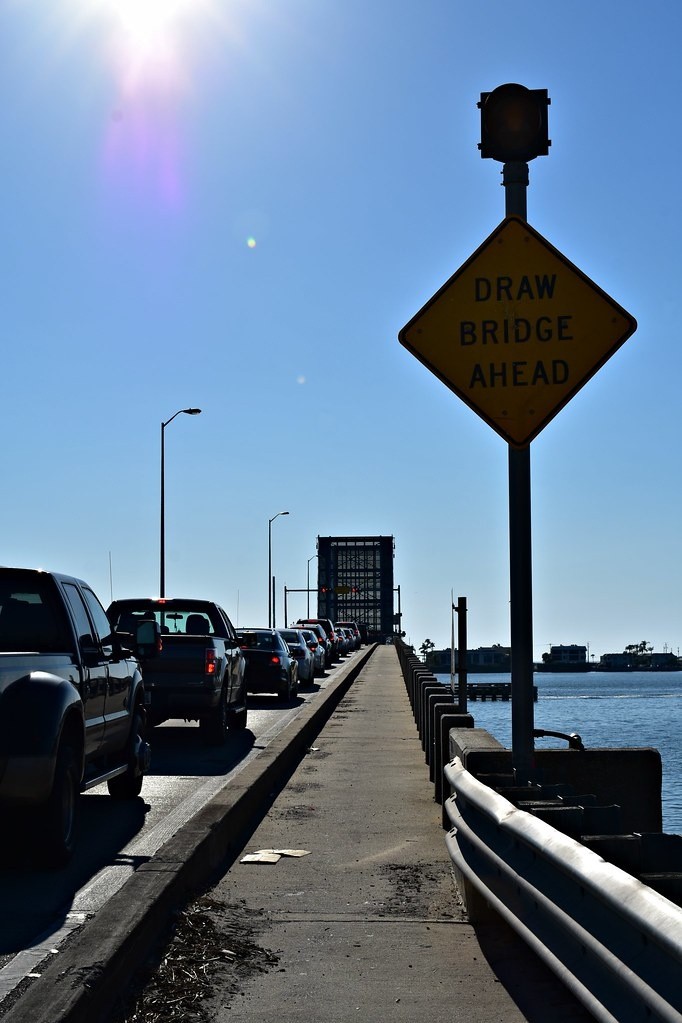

[352,587,364,592]
[475,81,552,160]
[321,587,333,593]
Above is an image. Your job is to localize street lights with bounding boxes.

[269,511,290,628]
[159,408,203,628]
[307,554,322,620]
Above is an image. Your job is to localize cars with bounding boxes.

[297,619,367,665]
[300,629,327,677]
[232,627,299,706]
[276,629,318,688]
[288,623,333,668]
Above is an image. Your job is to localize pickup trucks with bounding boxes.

[102,598,247,741]
[0,567,161,851]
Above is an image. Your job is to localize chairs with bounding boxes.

[186,615,209,636]
